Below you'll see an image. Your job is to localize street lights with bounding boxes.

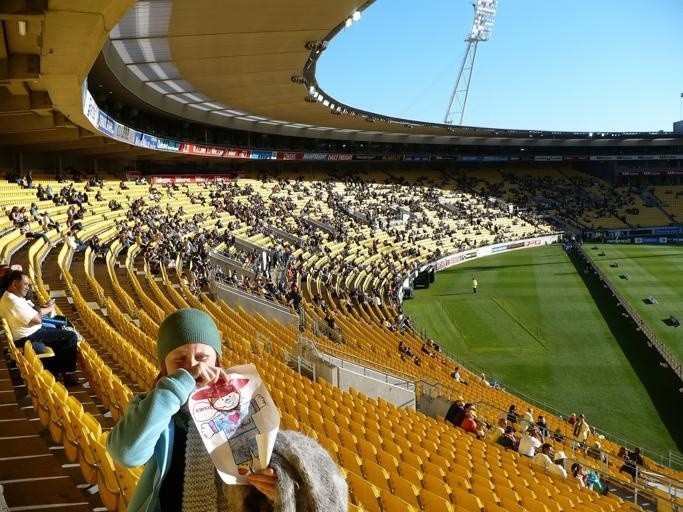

[443,1,499,127]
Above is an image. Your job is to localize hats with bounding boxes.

[155,308,221,362]
[552,449,567,461]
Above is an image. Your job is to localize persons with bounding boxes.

[450,367,646,495]
[107,308,279,511]
[1,265,85,387]
[8,169,683,365]
